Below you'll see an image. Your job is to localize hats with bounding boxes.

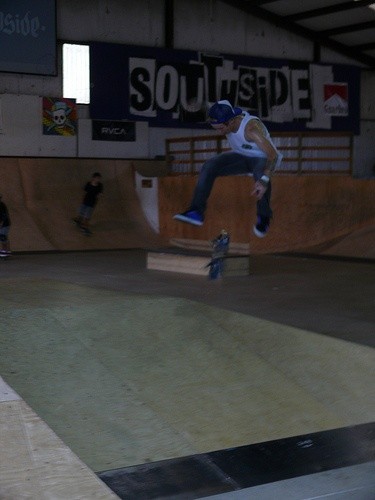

[207,100,242,125]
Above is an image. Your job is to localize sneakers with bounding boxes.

[173,210,203,226]
[0,251,12,257]
[253,215,269,238]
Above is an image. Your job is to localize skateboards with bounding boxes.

[72,215,96,240]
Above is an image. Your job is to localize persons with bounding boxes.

[0,193,13,257]
[72,172,103,234]
[173,98,284,237]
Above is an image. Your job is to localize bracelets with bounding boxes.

[261,175,270,183]
